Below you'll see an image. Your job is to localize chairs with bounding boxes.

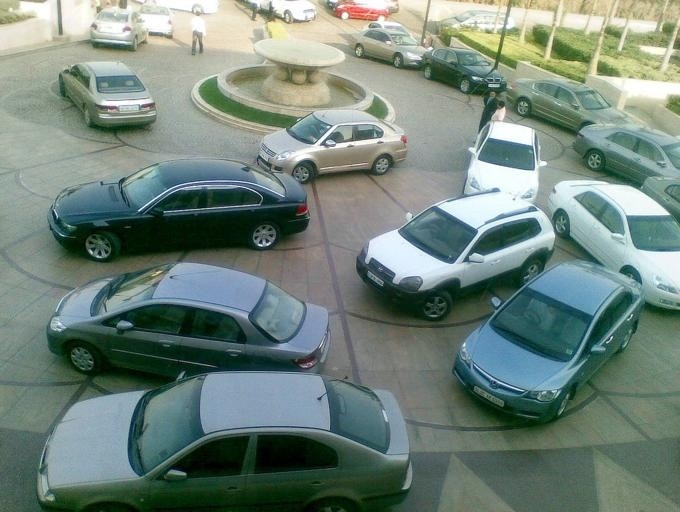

[559,315,587,347]
[99,82,109,88]
[124,80,134,86]
[525,298,556,332]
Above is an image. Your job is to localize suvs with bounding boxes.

[356,187,557,321]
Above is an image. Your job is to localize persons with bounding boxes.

[190,11,205,55]
[478,91,500,134]
[249,0,260,20]
[490,100,506,122]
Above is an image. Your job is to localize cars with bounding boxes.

[256,109,409,185]
[47,157,311,258]
[422,46,507,94]
[89,7,149,50]
[326,0,400,22]
[133,0,221,16]
[572,125,680,183]
[462,120,548,204]
[368,20,409,30]
[507,78,630,135]
[241,0,318,24]
[548,180,680,310]
[639,176,680,227]
[36,371,413,510]
[58,61,158,129]
[354,28,431,69]
[46,262,331,379]
[440,9,514,30]
[134,3,174,39]
[451,258,645,424]
